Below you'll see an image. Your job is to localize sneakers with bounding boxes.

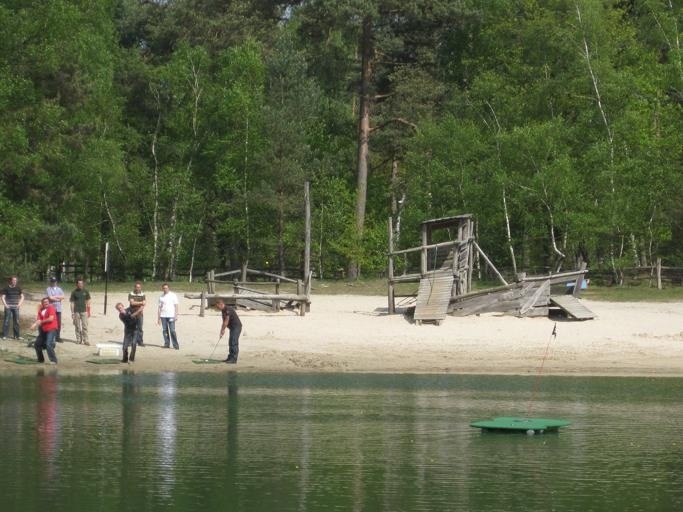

[138,341,143,346]
[161,346,179,349]
[2,337,24,340]
[55,338,63,343]
[33,360,55,365]
[222,359,235,363]
[76,341,89,345]
[120,360,133,365]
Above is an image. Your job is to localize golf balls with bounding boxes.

[266,262,269,265]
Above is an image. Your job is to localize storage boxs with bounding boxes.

[96,343,123,359]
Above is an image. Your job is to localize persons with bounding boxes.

[114,302,145,364]
[69,278,92,346]
[214,299,243,363]
[156,282,181,350]
[127,280,146,348]
[0,275,27,341]
[45,276,67,343]
[28,297,61,366]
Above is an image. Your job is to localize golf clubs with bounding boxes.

[205,336,222,362]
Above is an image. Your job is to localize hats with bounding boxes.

[50,277,55,282]
